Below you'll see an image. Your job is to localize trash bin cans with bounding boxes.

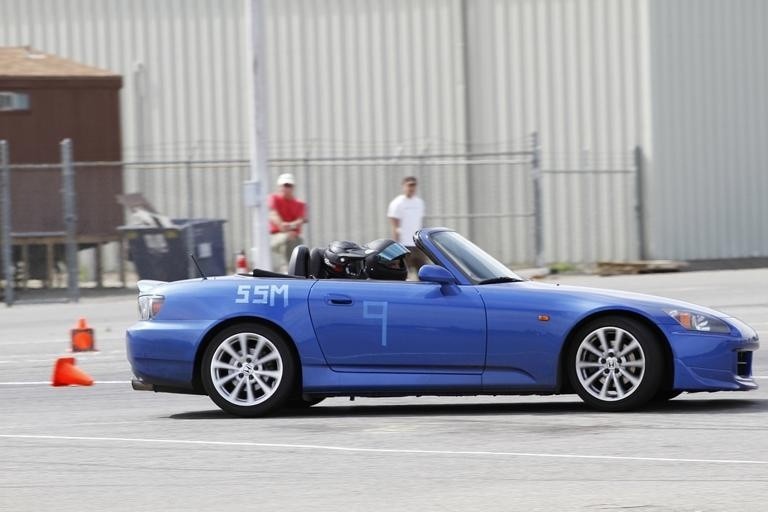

[117,218,229,282]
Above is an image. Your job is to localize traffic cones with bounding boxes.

[69,318,93,351]
[52,357,94,386]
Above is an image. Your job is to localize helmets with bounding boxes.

[366,239,411,280]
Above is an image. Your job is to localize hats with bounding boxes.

[278,173,295,185]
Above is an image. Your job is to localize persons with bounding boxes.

[388,175,427,272]
[266,172,309,275]
[361,239,412,281]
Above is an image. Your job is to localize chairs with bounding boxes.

[290,245,309,277]
[310,249,328,274]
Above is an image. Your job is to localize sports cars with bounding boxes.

[122,228,759,416]
